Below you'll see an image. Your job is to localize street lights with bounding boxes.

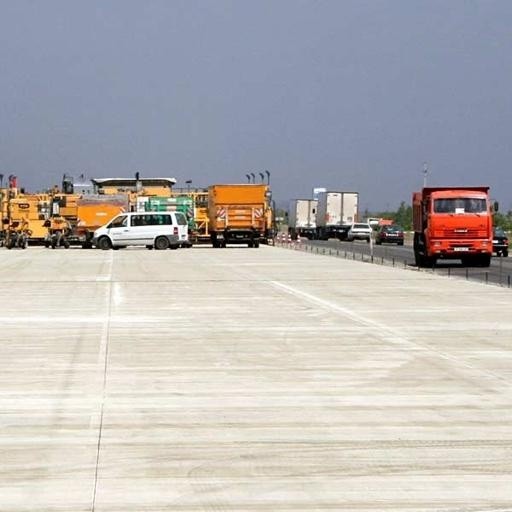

[246,170,270,186]
[423,160,429,187]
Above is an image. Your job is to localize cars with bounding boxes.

[347,216,405,246]
[491,230,510,256]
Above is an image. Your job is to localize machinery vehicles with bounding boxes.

[1,173,278,250]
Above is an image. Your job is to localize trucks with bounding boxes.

[412,185,495,268]
[314,190,358,241]
[287,199,316,240]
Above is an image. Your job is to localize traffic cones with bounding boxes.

[276,232,280,243]
[281,232,286,244]
[287,231,292,244]
[296,233,302,244]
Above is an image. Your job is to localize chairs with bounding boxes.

[133,216,171,225]
